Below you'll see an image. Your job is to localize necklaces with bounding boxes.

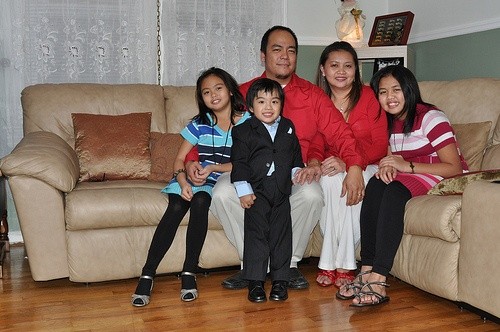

[393,132,405,158]
[212,115,232,177]
[330,92,349,112]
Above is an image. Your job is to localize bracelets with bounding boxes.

[172,168,187,182]
[409,161,415,174]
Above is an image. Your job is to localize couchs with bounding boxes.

[0,76,500,319]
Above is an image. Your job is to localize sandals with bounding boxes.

[316,270,336,287]
[131,276,154,306]
[336,270,372,300]
[349,282,390,307]
[335,270,356,289]
[180,271,198,301]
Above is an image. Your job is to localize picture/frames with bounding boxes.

[373,57,404,76]
[368,11,415,46]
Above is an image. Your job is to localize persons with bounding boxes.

[336,65,470,308]
[293,40,389,292]
[131,26,366,308]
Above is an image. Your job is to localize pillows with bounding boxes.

[452,121,492,171]
[149,132,182,184]
[426,169,500,196]
[71,111,152,185]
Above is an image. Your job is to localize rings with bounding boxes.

[357,193,362,197]
[331,166,335,170]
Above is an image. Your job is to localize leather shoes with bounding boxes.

[267,267,308,289]
[269,280,288,300]
[221,269,248,289]
[248,280,267,302]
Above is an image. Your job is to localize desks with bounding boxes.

[355,46,416,79]
